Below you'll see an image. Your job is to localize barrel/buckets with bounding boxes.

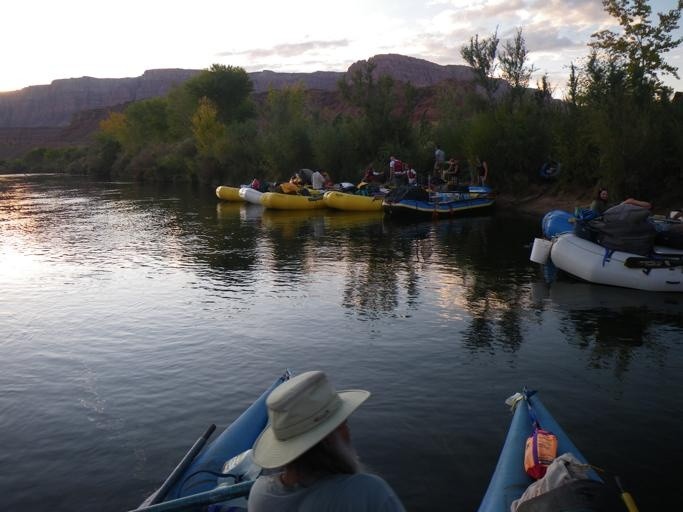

[529,238,552,265]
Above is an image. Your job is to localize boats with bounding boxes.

[212,183,241,203]
[146,365,290,512]
[540,159,560,180]
[236,202,262,222]
[547,231,683,295]
[255,191,326,210]
[321,210,385,232]
[215,200,241,218]
[237,186,261,205]
[320,189,382,212]
[535,209,577,244]
[471,382,639,512]
[376,194,497,220]
[259,206,325,230]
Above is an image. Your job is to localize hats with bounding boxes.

[251,368,371,468]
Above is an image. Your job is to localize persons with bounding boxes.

[590,188,609,217]
[247,370,406,512]
[620,194,651,209]
[244,143,491,196]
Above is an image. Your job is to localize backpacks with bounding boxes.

[392,160,403,172]
